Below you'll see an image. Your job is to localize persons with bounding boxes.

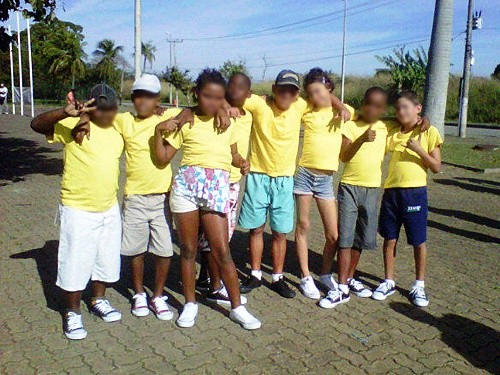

[224,70,351,298]
[295,68,398,298]
[155,68,262,330]
[319,86,431,309]
[73,73,231,321]
[372,90,444,307]
[0,84,9,114]
[30,84,167,340]
[173,72,254,305]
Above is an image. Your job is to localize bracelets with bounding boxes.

[416,146,420,152]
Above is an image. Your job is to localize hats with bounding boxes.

[131,73,161,94]
[275,69,300,89]
[88,83,117,106]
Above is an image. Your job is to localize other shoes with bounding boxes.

[196,277,210,291]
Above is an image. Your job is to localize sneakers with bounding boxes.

[411,285,429,306]
[176,302,199,327]
[240,274,262,293]
[131,292,150,317]
[63,301,87,340]
[88,296,122,322]
[148,296,174,321]
[371,279,396,301]
[348,278,372,297]
[319,273,338,291]
[299,275,320,299]
[318,288,350,309]
[271,279,296,298]
[229,305,261,329]
[206,284,247,304]
